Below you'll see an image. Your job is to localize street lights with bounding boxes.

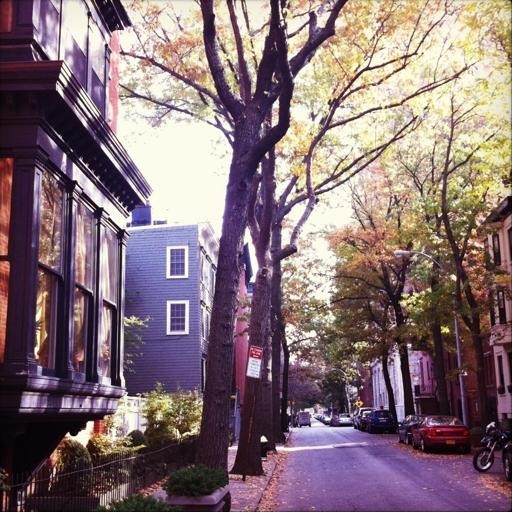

[394,249,466,423]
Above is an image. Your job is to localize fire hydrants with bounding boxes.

[259,435,269,461]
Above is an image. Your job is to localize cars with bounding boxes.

[396,413,429,445]
[412,414,470,452]
[297,411,312,427]
[352,406,394,434]
[313,413,353,426]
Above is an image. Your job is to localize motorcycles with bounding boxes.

[472,416,512,481]
